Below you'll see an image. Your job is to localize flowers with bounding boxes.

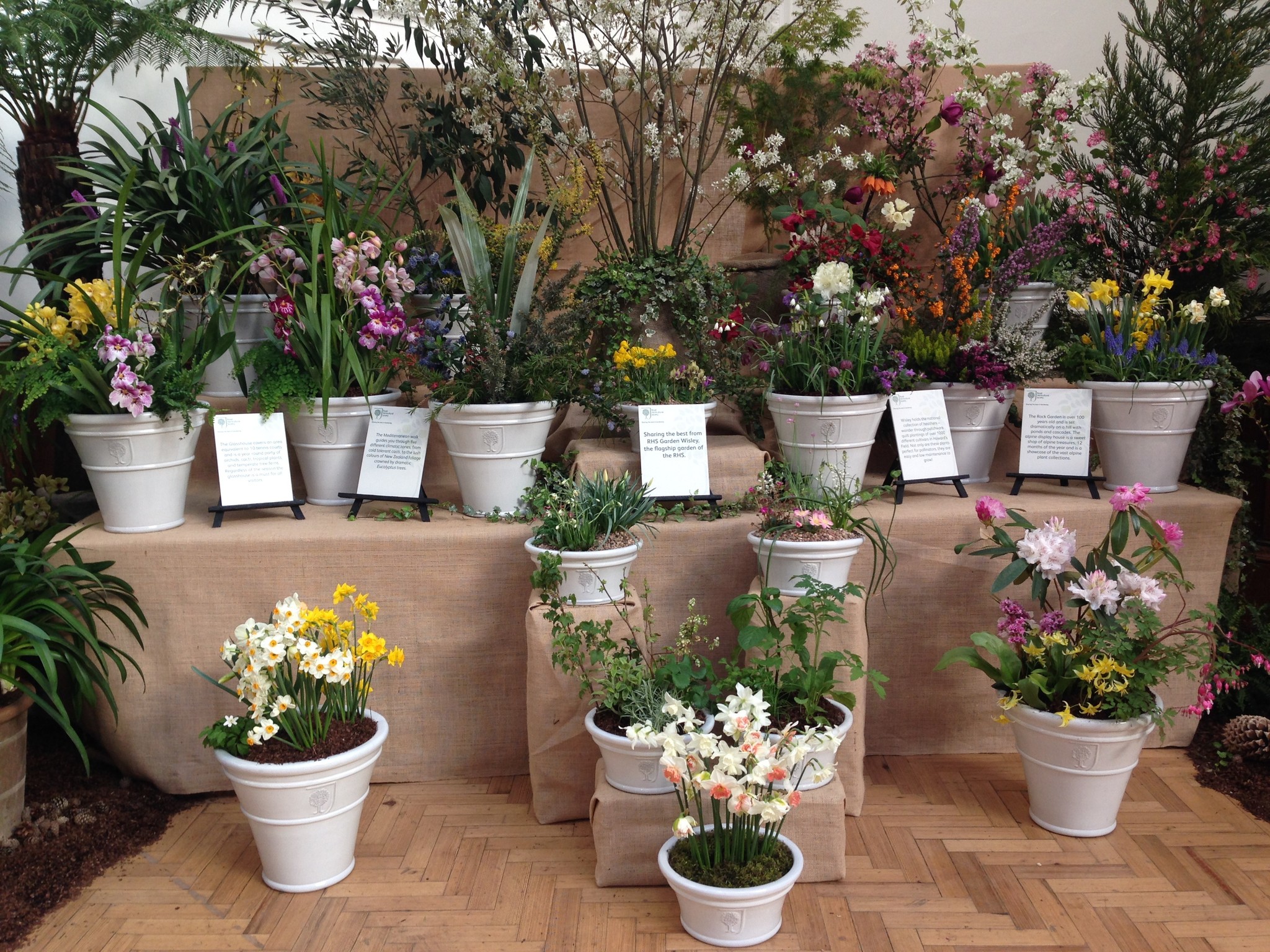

[0,0,1270,873]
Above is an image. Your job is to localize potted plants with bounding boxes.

[0,518,149,852]
[528,551,722,797]
[707,571,892,794]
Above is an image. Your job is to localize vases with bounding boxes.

[998,682,1169,842]
[763,388,891,510]
[210,703,388,896]
[922,380,1017,486]
[178,293,283,398]
[749,517,872,592]
[427,400,560,521]
[1002,279,1054,351]
[1075,377,1218,494]
[657,822,805,947]
[523,532,643,606]
[54,400,213,533]
[270,387,406,509]
[615,399,718,455]
